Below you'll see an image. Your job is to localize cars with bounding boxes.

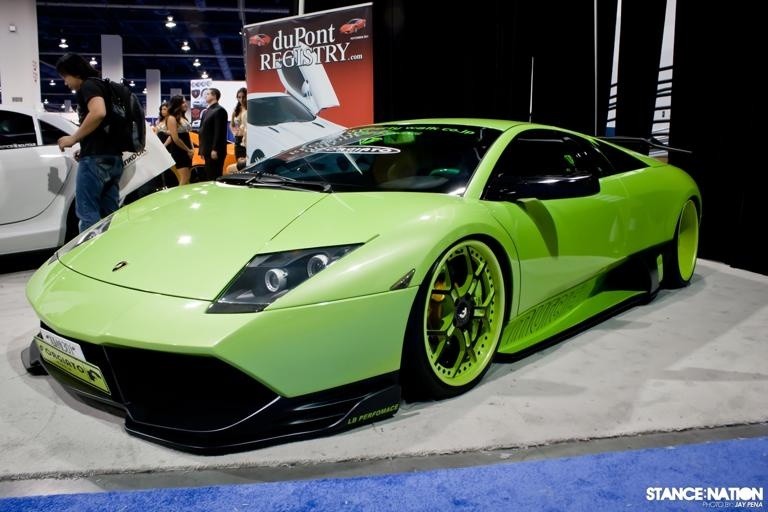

[150,128,239,185]
[248,33,272,46]
[0,103,176,257]
[338,18,366,35]
[193,96,208,108]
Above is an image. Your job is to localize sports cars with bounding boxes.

[248,39,349,166]
[19,117,705,461]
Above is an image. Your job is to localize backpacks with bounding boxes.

[80,76,147,153]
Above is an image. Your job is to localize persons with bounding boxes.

[151,88,247,185]
[55,51,125,235]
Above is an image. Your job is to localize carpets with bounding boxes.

[0,434,767,511]
[0,258,767,484]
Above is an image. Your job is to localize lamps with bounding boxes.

[57,12,212,96]
